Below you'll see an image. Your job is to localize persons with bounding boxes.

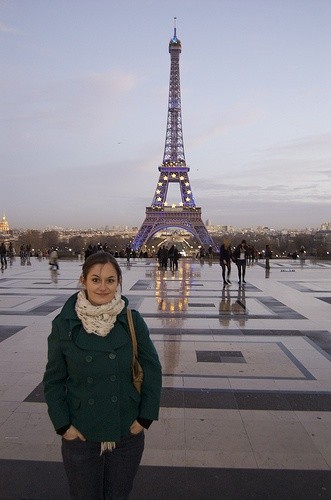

[247,244,263,262]
[287,247,306,260]
[219,237,234,285]
[232,239,249,284]
[41,251,162,500]
[36,245,60,271]
[0,243,7,265]
[157,245,180,271]
[198,244,214,259]
[264,245,273,269]
[8,240,32,260]
[83,242,156,263]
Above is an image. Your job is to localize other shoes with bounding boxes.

[224,279,246,285]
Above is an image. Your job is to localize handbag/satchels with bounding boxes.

[126,307,143,394]
